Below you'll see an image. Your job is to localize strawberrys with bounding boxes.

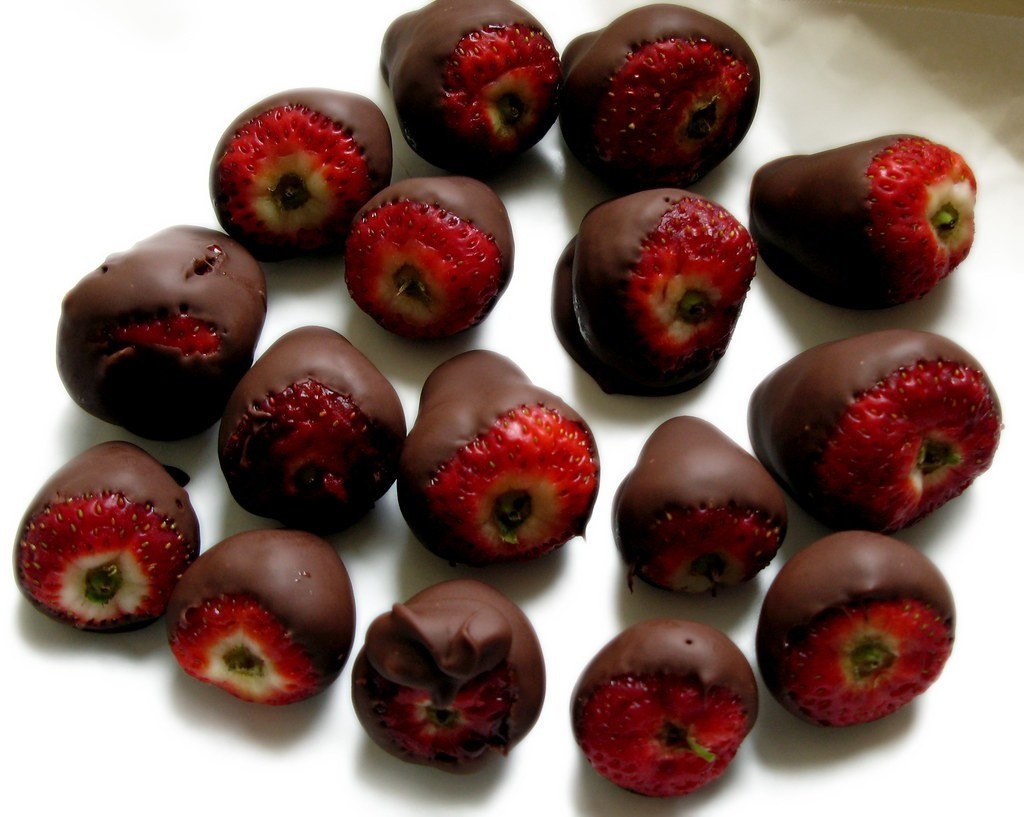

[12,0,1002,799]
